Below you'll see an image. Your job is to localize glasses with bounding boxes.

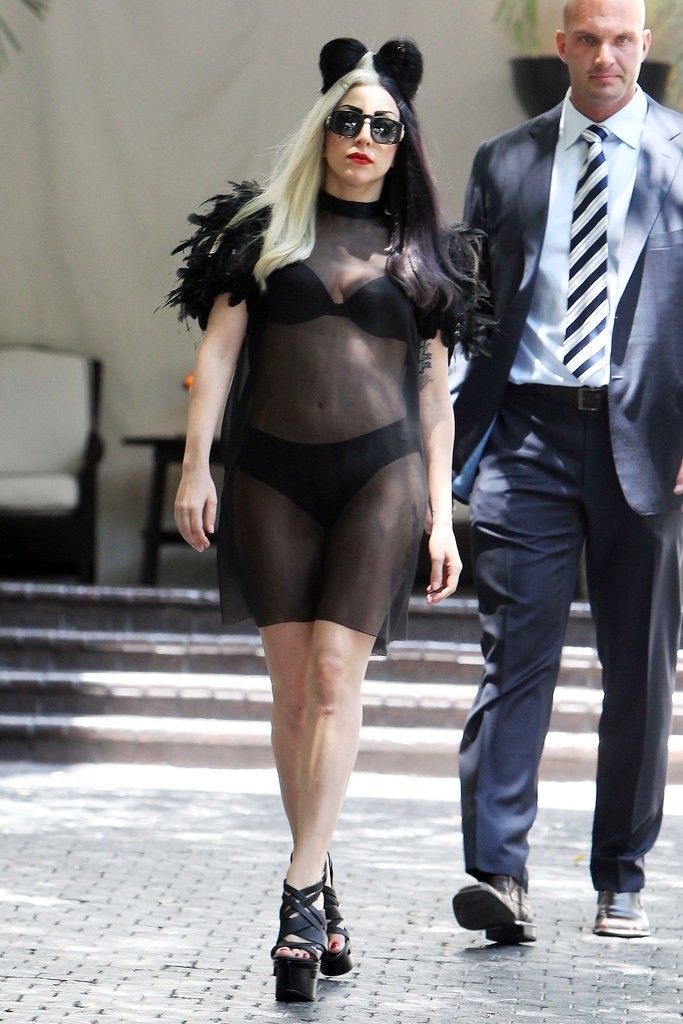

[325,109,405,145]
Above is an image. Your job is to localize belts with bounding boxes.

[509,377,611,411]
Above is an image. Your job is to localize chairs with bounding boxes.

[0,342,106,585]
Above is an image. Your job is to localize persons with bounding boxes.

[452,0,682,944]
[176,37,463,1002]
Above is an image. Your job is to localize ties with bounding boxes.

[563,123,612,384]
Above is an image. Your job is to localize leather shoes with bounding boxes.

[453,873,539,944]
[593,887,652,936]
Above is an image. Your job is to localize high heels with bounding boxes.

[271,859,328,1004]
[290,848,353,978]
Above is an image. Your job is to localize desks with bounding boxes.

[121,434,223,588]
